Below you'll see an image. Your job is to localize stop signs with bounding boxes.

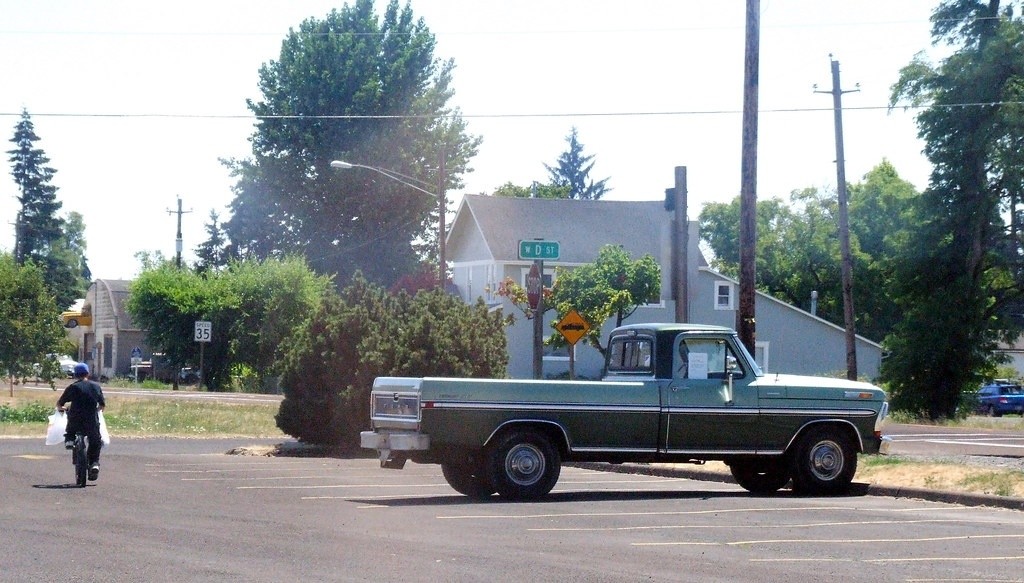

[526,262,542,312]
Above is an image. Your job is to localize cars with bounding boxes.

[33,352,81,378]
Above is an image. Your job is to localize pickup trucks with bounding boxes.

[359,320,888,503]
[128,351,200,383]
[973,379,1024,418]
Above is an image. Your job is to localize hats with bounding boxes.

[74,363,89,375]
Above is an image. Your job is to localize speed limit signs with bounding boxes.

[194,319,212,342]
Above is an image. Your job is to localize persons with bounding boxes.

[56,362,106,481]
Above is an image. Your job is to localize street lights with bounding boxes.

[329,142,447,292]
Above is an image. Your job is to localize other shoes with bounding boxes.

[88,464,100,481]
[65,440,74,451]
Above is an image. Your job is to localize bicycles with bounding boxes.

[58,403,107,486]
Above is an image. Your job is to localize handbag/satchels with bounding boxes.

[98,408,110,447]
[46,408,67,446]
